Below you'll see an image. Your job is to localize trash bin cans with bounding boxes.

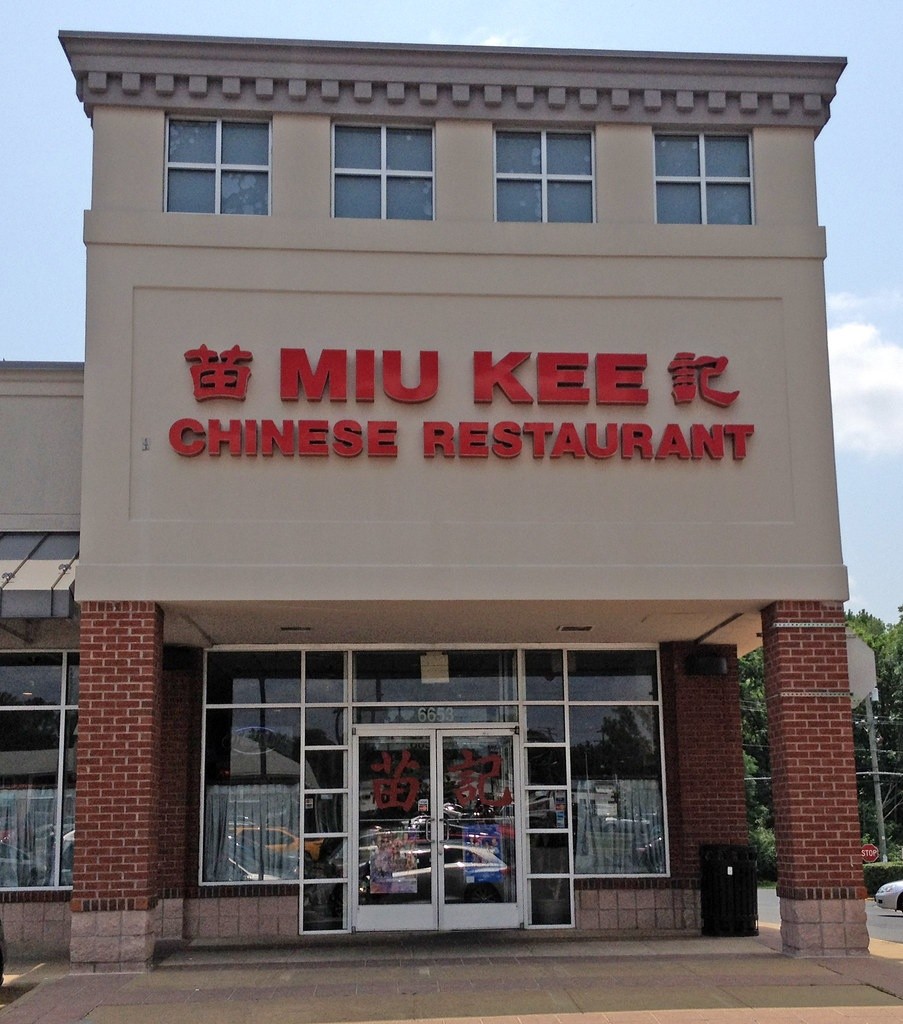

[700,842,760,937]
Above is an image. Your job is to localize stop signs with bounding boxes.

[861,844,880,863]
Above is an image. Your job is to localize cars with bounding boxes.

[630,836,666,873]
[0,814,75,887]
[602,812,653,833]
[228,804,565,915]
[874,880,903,912]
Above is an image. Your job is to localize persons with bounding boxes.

[465,838,500,864]
[370,834,416,894]
